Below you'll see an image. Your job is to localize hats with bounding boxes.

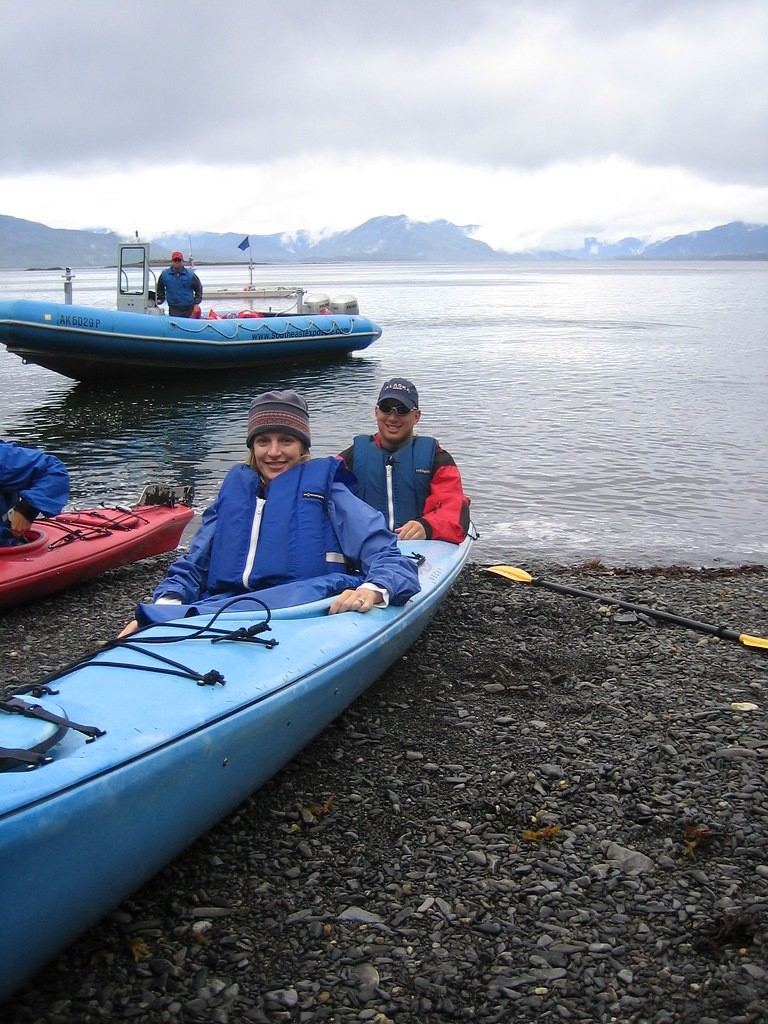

[377,378,418,412]
[171,251,183,261]
[246,390,311,448]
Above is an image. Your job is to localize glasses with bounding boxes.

[172,259,181,262]
[376,402,417,415]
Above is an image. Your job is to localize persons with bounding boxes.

[0,439,70,547]
[336,378,472,543]
[116,390,422,637]
[157,251,203,318]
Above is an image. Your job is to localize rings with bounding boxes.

[356,598,365,605]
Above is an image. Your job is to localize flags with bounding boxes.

[238,236,250,251]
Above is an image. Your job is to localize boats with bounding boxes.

[0,517,481,1007]
[0,242,384,380]
[0,485,196,610]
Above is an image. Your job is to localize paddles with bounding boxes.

[480,564,768,652]
